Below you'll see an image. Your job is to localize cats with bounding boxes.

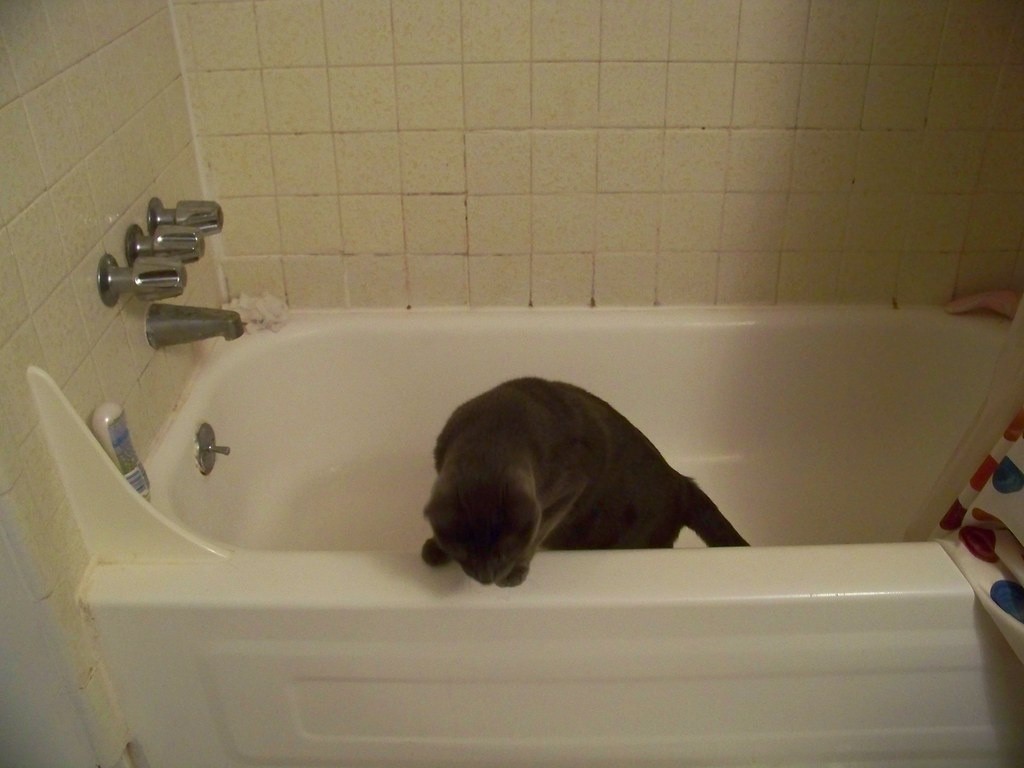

[419,375,753,588]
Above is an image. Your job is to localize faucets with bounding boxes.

[146,303,244,350]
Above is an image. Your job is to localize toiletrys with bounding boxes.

[91,400,151,503]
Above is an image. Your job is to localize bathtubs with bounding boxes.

[79,308,1023,762]
